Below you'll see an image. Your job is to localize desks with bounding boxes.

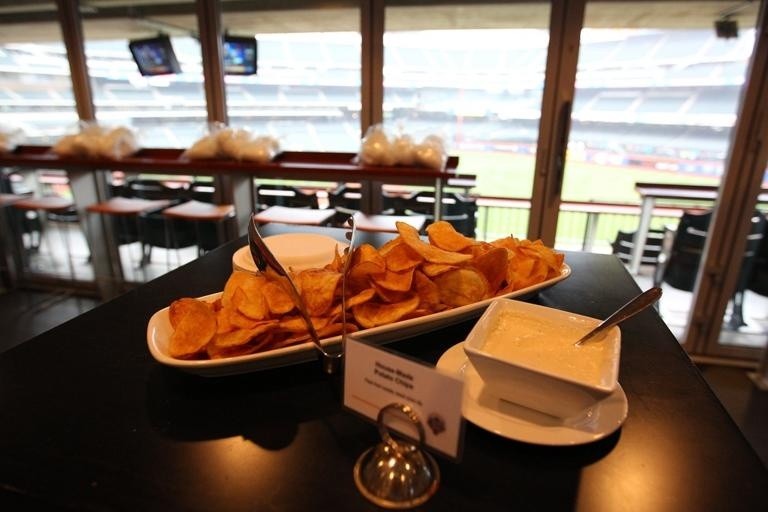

[0,221,766,512]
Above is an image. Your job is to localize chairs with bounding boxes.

[1,178,476,275]
[605,205,767,330]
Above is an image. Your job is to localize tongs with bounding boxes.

[249,211,357,378]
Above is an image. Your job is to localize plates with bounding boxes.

[145,234,573,380]
[230,239,354,274]
[434,339,629,447]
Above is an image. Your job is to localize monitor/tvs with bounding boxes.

[129,34,182,76]
[223,35,258,76]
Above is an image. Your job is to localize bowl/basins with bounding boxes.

[461,295,625,420]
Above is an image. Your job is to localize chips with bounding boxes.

[168,220,564,359]
